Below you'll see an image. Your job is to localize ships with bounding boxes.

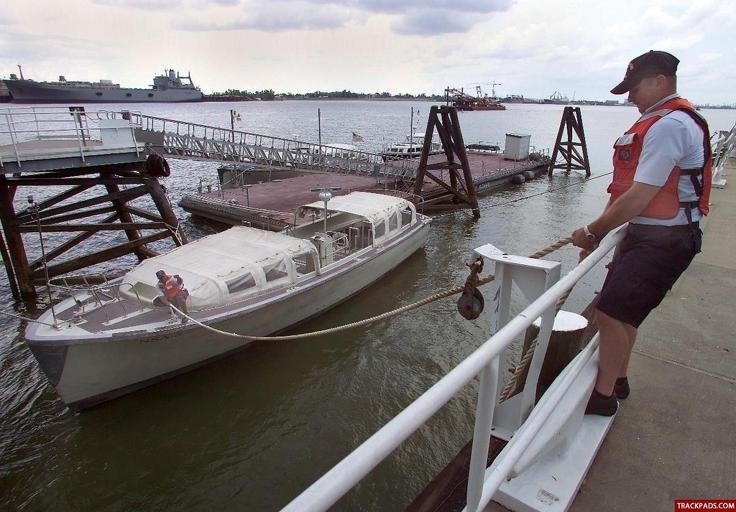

[2,64,202,104]
[445,80,508,111]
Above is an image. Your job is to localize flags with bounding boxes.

[353,133,364,142]
[232,112,242,121]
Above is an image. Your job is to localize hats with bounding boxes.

[610,50,680,95]
[156,270,165,278]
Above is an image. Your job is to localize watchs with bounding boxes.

[583,225,599,242]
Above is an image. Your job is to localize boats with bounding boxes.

[382,108,446,161]
[239,108,383,168]
[19,184,433,410]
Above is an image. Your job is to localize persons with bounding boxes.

[572,49,712,415]
[152,270,189,323]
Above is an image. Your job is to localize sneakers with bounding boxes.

[182,317,188,323]
[584,376,630,417]
